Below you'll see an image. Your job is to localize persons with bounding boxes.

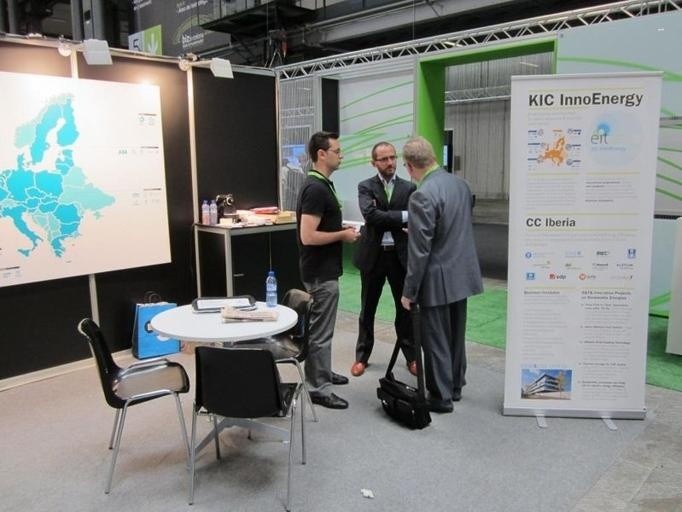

[280,158,306,210]
[401,135,485,414]
[295,130,362,409]
[351,140,418,377]
[299,153,314,176]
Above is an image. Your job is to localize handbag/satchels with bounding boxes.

[132,291,181,360]
[377,376,431,430]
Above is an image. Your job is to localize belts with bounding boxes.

[381,245,394,251]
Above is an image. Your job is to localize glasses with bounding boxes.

[328,148,340,153]
[376,155,395,161]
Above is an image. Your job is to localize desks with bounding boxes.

[151,300,300,460]
[194,211,301,298]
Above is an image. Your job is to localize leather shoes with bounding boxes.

[331,372,348,384]
[407,361,417,376]
[312,393,348,409]
[351,362,368,376]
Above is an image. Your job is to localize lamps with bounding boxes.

[177,58,233,79]
[58,39,112,65]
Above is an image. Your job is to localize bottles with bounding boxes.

[209,200,218,226]
[265,271,279,308]
[201,200,211,225]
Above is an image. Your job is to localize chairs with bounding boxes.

[189,345,305,511]
[222,288,320,440]
[78,318,192,495]
